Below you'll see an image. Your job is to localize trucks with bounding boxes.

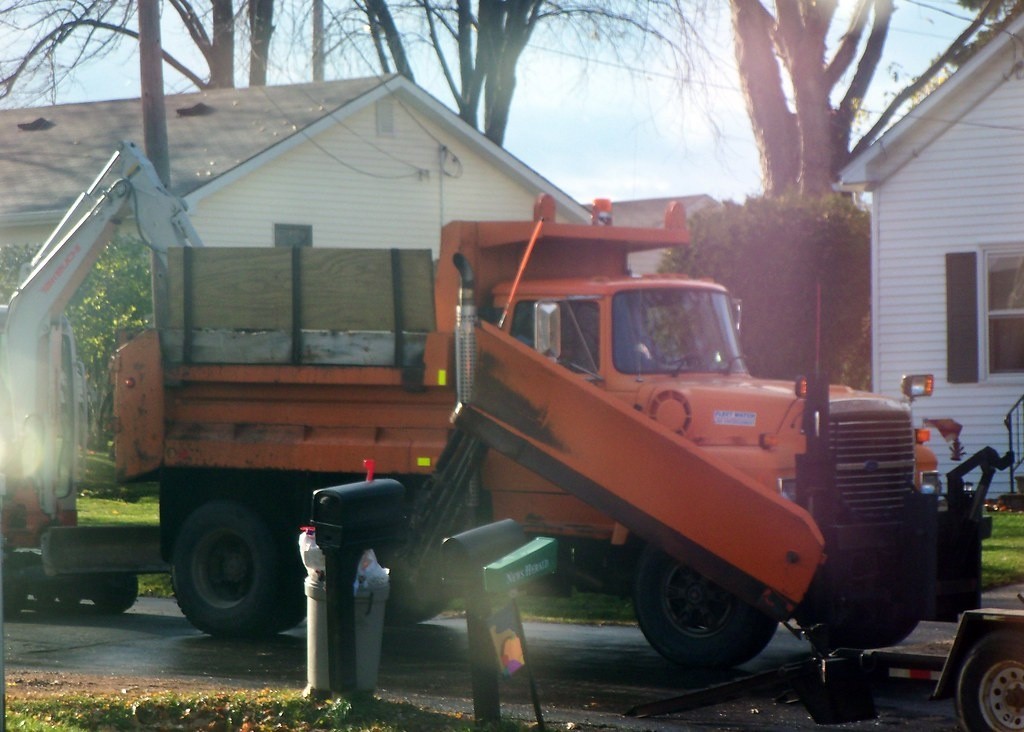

[113,194,1014,675]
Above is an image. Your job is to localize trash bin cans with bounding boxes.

[303,574,391,696]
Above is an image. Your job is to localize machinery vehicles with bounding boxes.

[0,138,206,612]
